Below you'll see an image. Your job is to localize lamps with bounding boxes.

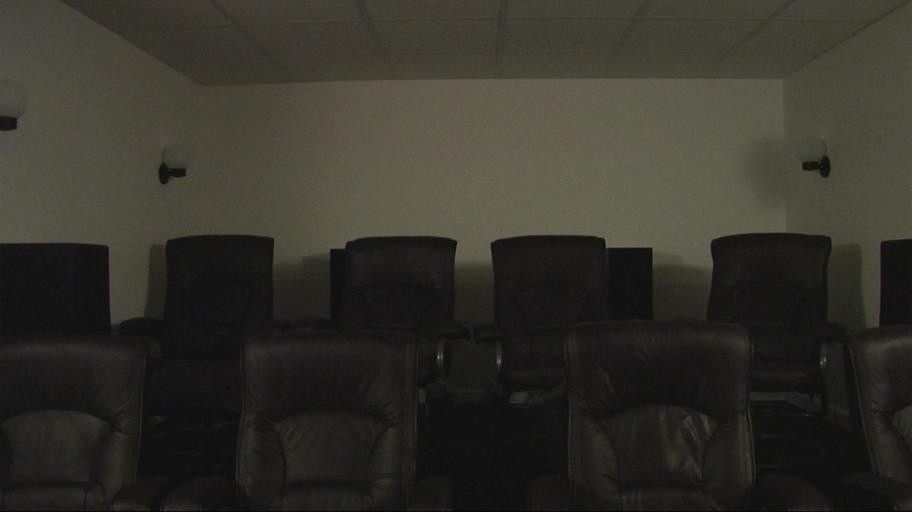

[2,78,28,130]
[797,137,832,179]
[159,145,190,186]
